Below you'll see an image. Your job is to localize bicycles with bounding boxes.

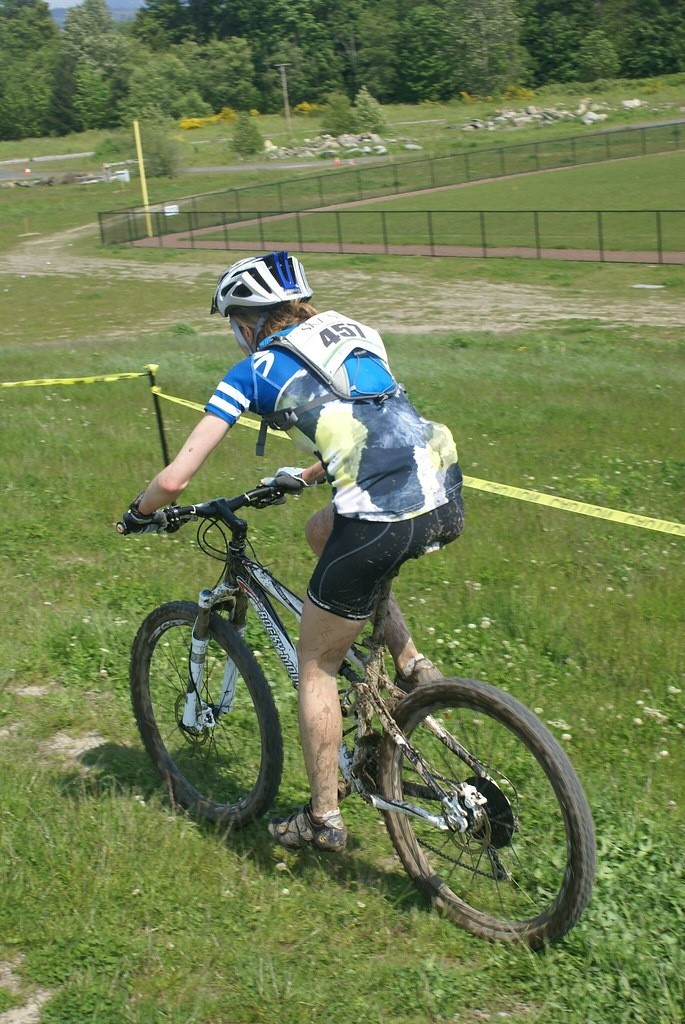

[113,471,601,951]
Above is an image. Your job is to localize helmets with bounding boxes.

[210,251,314,317]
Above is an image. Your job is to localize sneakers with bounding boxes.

[394,657,444,693]
[268,797,347,851]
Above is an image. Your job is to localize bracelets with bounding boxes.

[128,491,156,519]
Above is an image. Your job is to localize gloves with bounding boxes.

[261,466,317,495]
[123,491,167,534]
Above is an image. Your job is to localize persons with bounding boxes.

[123,250,465,855]
